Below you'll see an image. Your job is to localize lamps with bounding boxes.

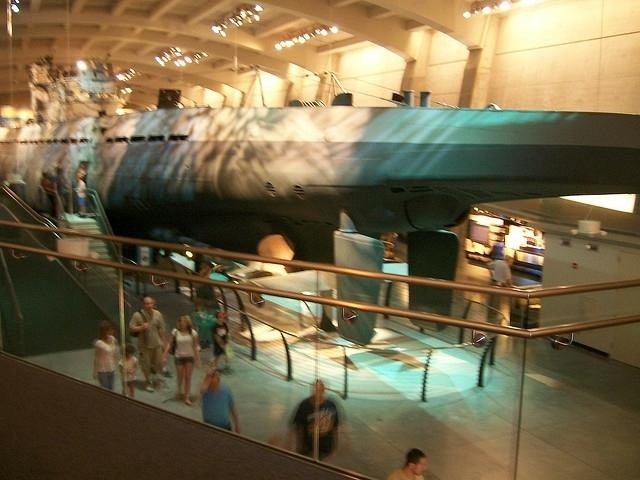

[116,0,518,86]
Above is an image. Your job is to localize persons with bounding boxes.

[489,232,506,260]
[189,302,233,374]
[198,261,224,312]
[487,255,514,324]
[293,378,340,464]
[201,362,241,433]
[385,449,427,480]
[41,161,88,220]
[94,296,202,406]
